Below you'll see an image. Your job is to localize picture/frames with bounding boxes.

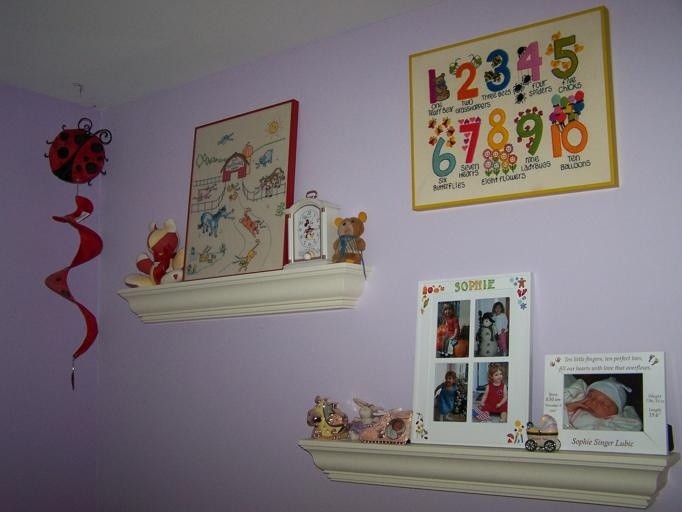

[409,272,531,449]
[182,98,299,282]
[409,5,620,213]
[543,352,667,455]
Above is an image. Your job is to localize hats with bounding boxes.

[584,379,632,416]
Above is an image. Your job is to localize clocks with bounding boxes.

[284,196,340,265]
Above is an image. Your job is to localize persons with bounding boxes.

[489,300,509,357]
[435,369,462,421]
[475,363,508,423]
[439,302,462,355]
[566,379,619,421]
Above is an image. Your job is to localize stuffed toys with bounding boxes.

[122,218,185,289]
[331,210,368,265]
